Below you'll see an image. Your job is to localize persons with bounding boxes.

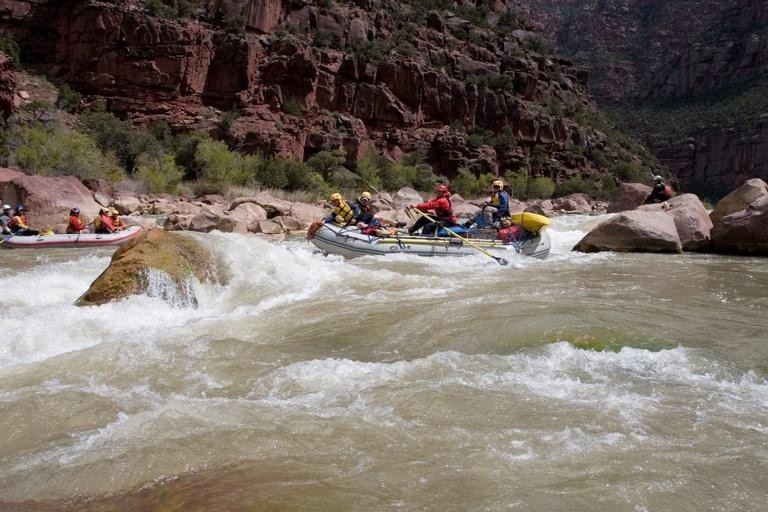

[0,198,126,235]
[397,184,457,238]
[640,174,673,208]
[479,182,515,231]
[320,191,373,235]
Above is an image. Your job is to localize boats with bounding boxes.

[307,219,550,261]
[0,226,143,248]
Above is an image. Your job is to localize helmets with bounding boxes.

[330,193,342,203]
[493,181,503,190]
[362,192,371,199]
[437,185,447,192]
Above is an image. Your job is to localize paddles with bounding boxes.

[411,206,508,266]
